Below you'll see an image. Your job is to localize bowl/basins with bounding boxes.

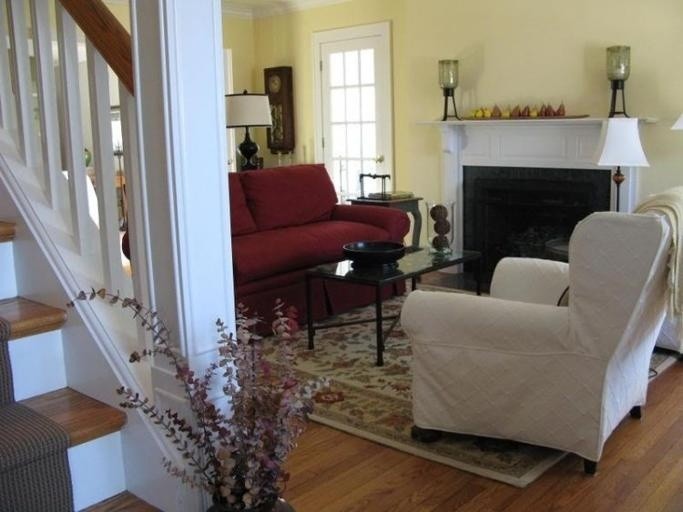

[342,239,406,263]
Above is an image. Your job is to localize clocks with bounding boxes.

[263,67,295,155]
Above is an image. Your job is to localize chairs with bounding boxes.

[400,209,672,477]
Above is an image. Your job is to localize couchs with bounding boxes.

[229,164,410,345]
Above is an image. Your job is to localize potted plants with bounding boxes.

[64,287,331,512]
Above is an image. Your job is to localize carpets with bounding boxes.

[238,277,680,488]
[0,317,75,512]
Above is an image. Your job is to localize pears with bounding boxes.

[471,101,565,118]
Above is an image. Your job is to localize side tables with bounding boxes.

[345,196,425,283]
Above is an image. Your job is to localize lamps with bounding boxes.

[597,117,651,213]
[224,89,273,170]
